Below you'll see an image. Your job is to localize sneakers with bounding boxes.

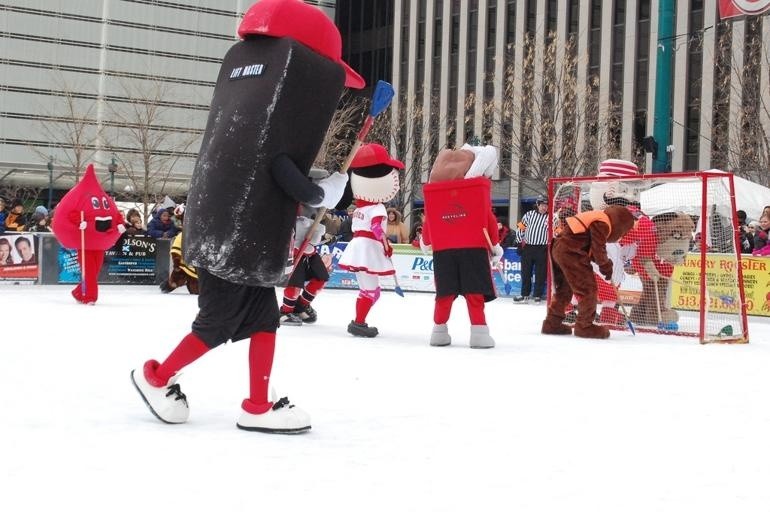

[514,295,541,303]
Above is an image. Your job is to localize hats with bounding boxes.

[11,199,48,224]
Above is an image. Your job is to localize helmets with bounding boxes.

[536,194,548,205]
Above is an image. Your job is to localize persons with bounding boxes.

[1,198,185,237]
[320,200,517,246]
[516,194,548,302]
[0,236,35,265]
[559,197,770,257]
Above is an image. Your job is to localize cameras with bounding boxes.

[740,226,746,232]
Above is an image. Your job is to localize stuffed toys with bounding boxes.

[561,159,642,327]
[130,1,367,434]
[629,211,694,325]
[335,143,405,339]
[279,166,328,324]
[541,205,635,338]
[50,162,127,306]
[419,143,504,349]
[159,205,200,294]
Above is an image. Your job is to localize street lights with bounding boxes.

[46,156,55,211]
[107,155,117,199]
